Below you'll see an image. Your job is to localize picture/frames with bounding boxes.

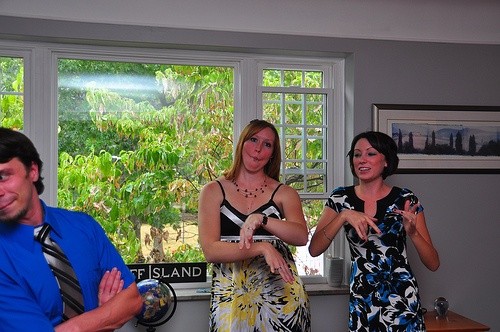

[370,102,500,175]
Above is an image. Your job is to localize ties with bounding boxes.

[34,222,85,324]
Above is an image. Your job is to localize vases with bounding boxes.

[326,257,344,287]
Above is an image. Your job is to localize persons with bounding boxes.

[0,127,144,332]
[308,130,439,332]
[198,119,312,332]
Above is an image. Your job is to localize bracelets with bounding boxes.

[260,212,268,225]
[323,227,332,241]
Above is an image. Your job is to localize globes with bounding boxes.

[134,276,178,332]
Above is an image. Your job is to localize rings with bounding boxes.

[248,228,251,230]
[252,228,255,230]
[402,210,404,213]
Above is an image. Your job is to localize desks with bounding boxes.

[424,310,491,332]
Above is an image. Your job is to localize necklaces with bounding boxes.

[231,175,267,198]
